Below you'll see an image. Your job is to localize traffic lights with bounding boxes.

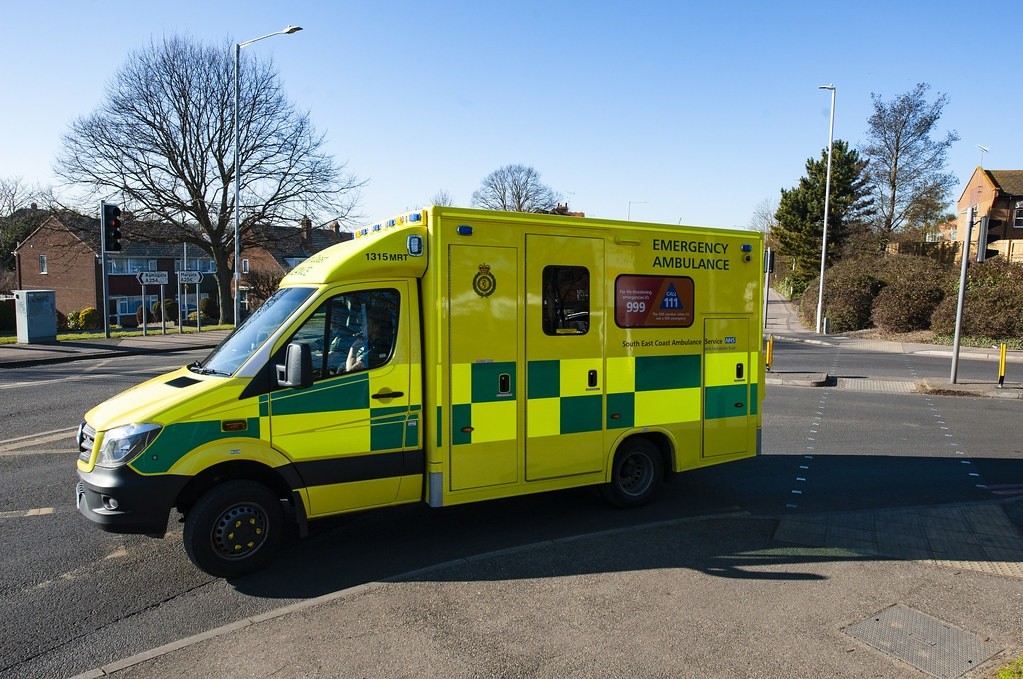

[104,204,123,253]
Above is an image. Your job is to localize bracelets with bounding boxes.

[350,346,357,350]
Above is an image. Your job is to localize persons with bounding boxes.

[337,306,391,377]
[308,308,354,370]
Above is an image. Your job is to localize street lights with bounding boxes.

[232,24,303,330]
[815,82,836,337]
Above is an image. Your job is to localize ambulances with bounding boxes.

[74,201,776,583]
